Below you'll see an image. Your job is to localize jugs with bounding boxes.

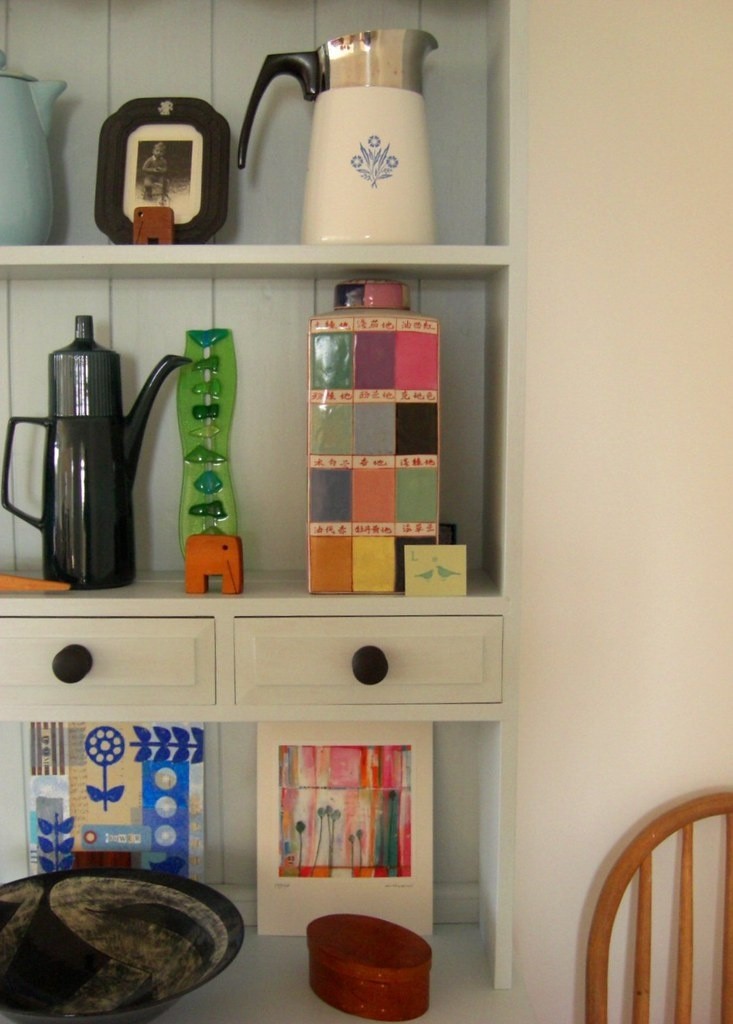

[0,52,66,246]
[238,29,439,246]
[0,315,193,590]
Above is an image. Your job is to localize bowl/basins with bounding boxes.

[0,867,244,1024]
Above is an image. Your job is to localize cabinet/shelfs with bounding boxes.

[0,0,528,989]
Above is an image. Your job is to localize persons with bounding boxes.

[143,142,169,205]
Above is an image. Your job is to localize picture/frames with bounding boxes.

[95,97,230,244]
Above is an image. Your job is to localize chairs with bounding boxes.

[584,791,733,1024]
